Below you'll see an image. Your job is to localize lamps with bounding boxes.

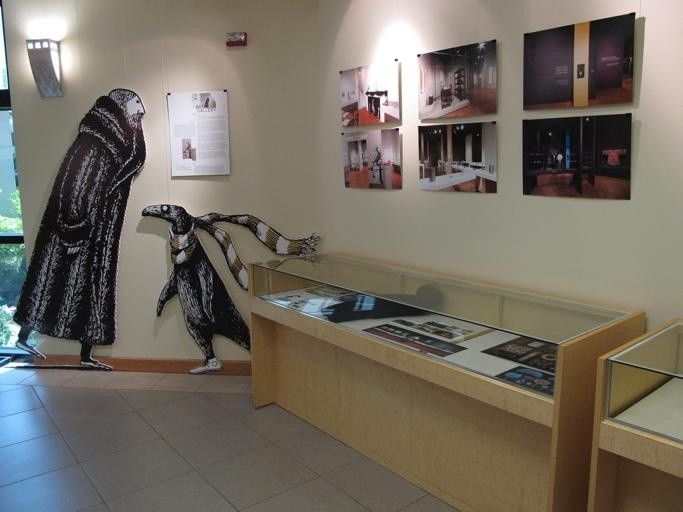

[26,38,64,99]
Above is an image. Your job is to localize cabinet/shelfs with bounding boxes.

[248,250,650,509]
[584,315,683,512]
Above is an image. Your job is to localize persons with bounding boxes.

[12,87,147,371]
